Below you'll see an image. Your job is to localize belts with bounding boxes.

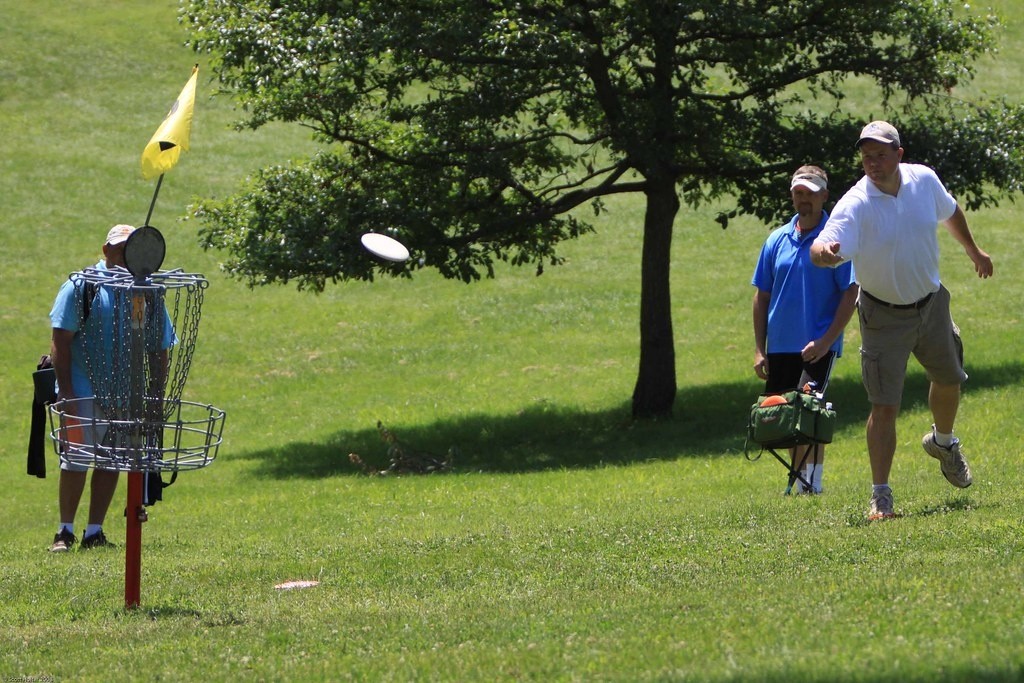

[860,287,932,310]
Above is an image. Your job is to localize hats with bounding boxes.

[105,224,135,245]
[790,173,828,192]
[855,120,901,149]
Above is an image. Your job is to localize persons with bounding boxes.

[809,120,993,519]
[48,224,181,554]
[751,162,858,499]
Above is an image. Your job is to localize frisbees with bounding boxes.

[759,395,788,405]
[361,232,409,262]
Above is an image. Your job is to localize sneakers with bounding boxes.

[869,485,895,517]
[51,526,76,553]
[920,423,972,488]
[79,527,120,549]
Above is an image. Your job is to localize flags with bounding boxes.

[140,67,202,179]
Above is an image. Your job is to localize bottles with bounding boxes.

[808,381,833,411]
